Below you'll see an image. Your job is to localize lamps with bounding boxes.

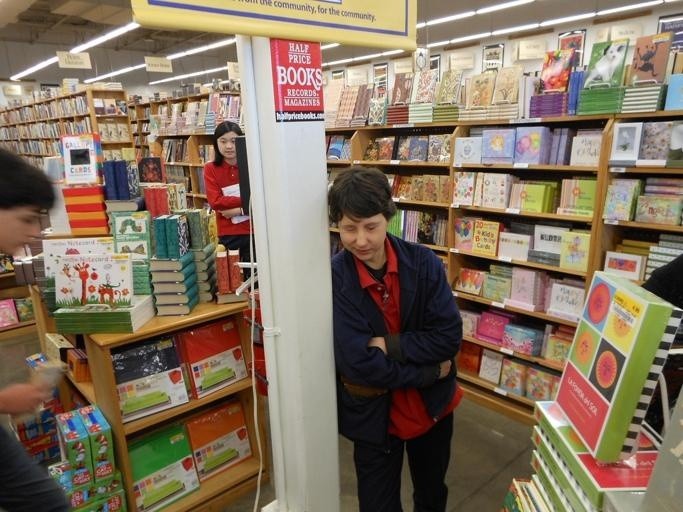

[321,43,404,68]
[9,22,236,81]
[416,1,662,49]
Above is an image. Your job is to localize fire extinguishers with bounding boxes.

[233,261,268,396]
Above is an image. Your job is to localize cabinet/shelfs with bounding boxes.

[443,114,616,410]
[325,125,357,254]
[0,88,266,511]
[585,109,683,287]
[352,120,462,279]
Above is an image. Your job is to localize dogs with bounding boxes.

[584,43,625,88]
